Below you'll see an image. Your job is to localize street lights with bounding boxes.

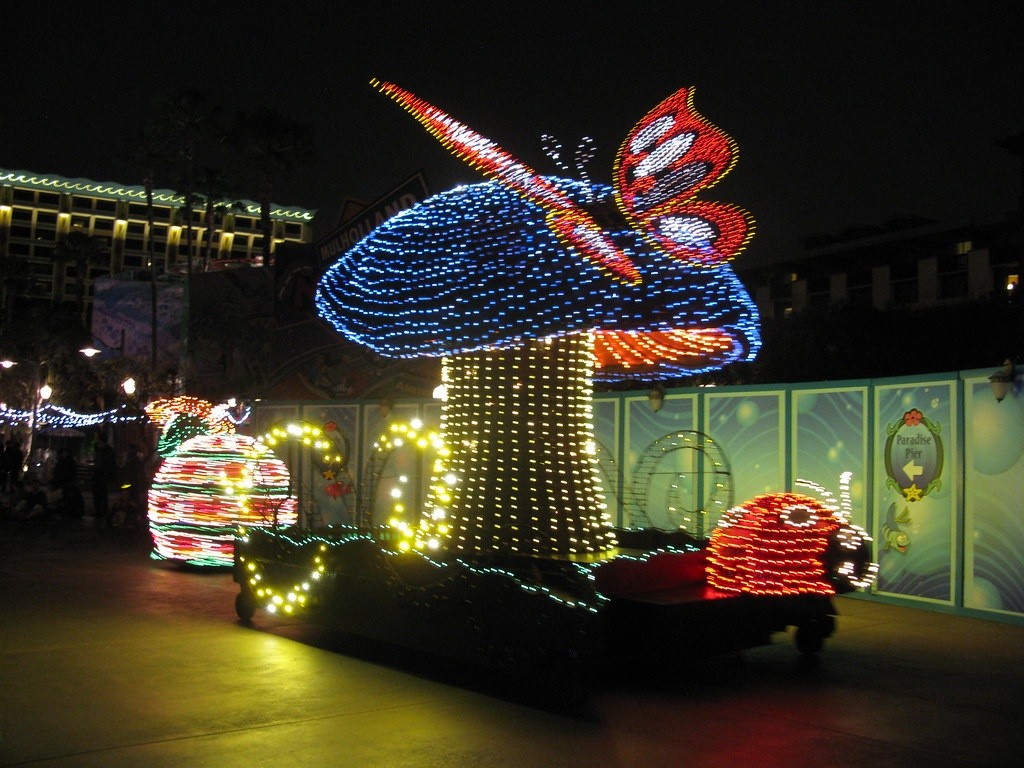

[1,360,36,431]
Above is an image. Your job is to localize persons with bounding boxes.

[0,431,141,518]
